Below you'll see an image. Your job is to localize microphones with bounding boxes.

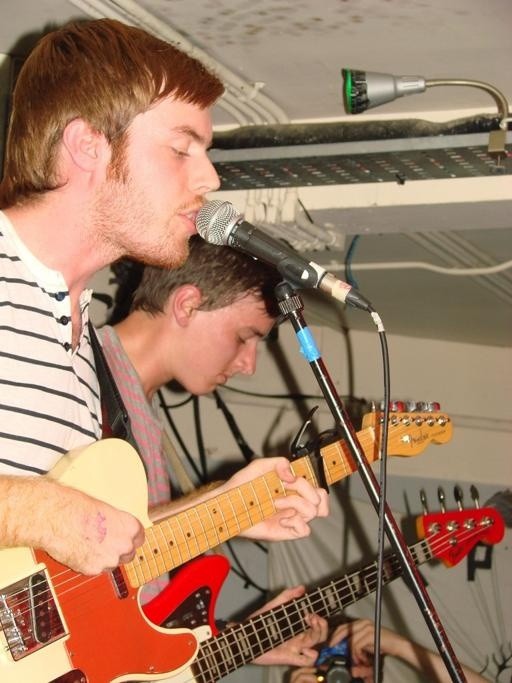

[191,200,372,311]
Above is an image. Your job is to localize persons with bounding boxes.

[0,20,320,683]
[281,619,497,683]
[86,228,327,683]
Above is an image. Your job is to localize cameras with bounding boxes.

[316,639,364,683]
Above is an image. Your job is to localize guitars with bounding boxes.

[0,401,452,683]
[141,479,503,683]
